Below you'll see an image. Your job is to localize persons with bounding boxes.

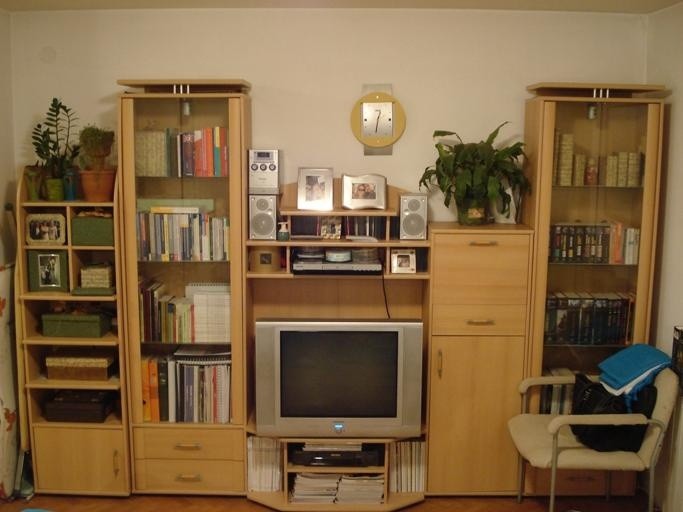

[40,256,59,285]
[398,257,408,267]
[352,184,375,199]
[30,221,58,239]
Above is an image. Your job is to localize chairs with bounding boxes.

[507,360,680,512]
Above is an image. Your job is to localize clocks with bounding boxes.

[349,83,407,155]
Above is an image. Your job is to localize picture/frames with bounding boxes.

[28,251,69,292]
[391,249,419,275]
[296,166,335,211]
[341,172,389,211]
[25,213,68,248]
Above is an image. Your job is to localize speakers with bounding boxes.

[399,194,428,240]
[248,195,278,241]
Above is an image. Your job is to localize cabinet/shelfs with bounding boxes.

[245,177,429,278]
[525,83,668,496]
[117,78,252,498]
[430,221,533,495]
[16,165,131,499]
[247,407,428,512]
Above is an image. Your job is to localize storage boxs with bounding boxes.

[41,314,110,339]
[45,352,116,381]
[44,394,116,424]
[72,217,115,249]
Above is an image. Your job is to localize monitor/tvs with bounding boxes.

[253,316,422,442]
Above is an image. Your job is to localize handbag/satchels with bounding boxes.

[598,343,673,397]
[570,374,622,452]
[622,385,656,452]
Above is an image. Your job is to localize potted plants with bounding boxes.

[79,126,117,203]
[418,121,533,226]
[32,97,79,202]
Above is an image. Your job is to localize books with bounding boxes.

[343,215,386,240]
[135,122,231,426]
[248,438,427,504]
[539,131,646,413]
